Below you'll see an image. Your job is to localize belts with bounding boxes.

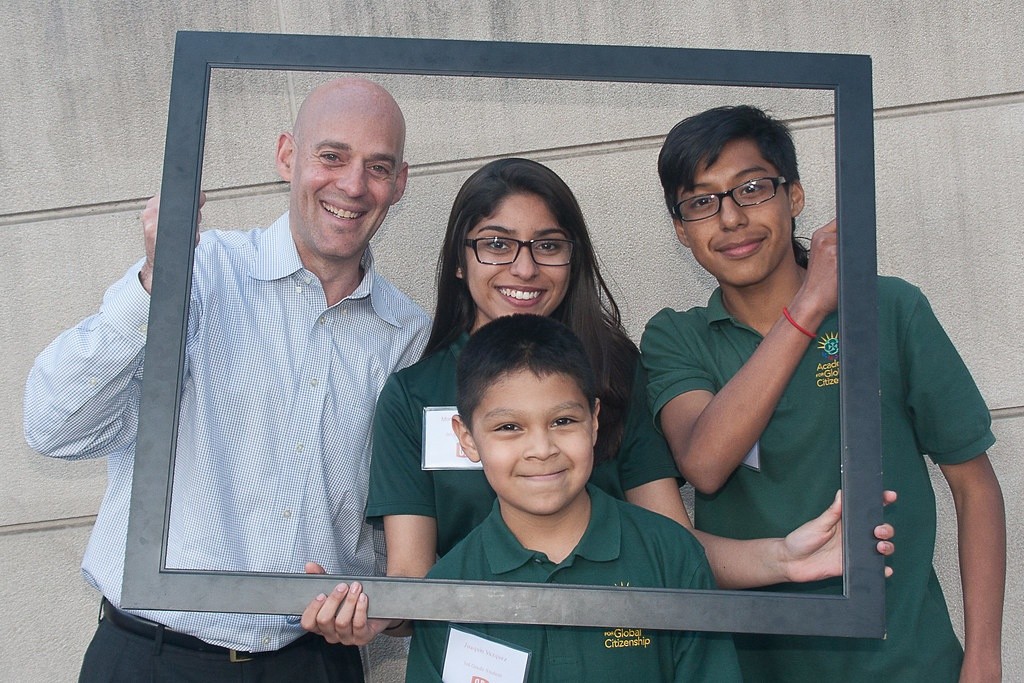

[103,598,319,662]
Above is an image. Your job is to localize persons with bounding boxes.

[405,315,744,683]
[303,159,896,682]
[23,79,434,683]
[643,108,1006,683]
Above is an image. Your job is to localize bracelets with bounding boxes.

[783,307,817,340]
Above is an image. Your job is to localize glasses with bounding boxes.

[464,237,576,266]
[672,175,796,222]
